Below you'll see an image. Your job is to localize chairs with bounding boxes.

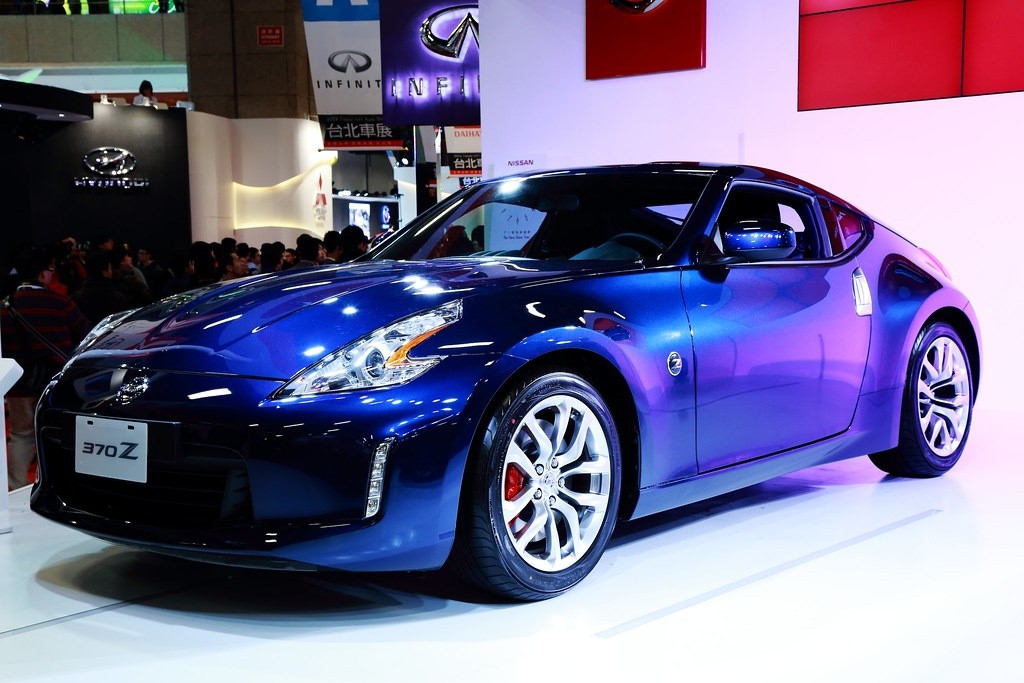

[723,225,797,261]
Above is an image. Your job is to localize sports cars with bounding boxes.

[29,161,986,605]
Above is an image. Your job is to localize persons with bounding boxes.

[0,248,91,490]
[13,226,372,327]
[133,80,158,106]
[429,225,484,259]
